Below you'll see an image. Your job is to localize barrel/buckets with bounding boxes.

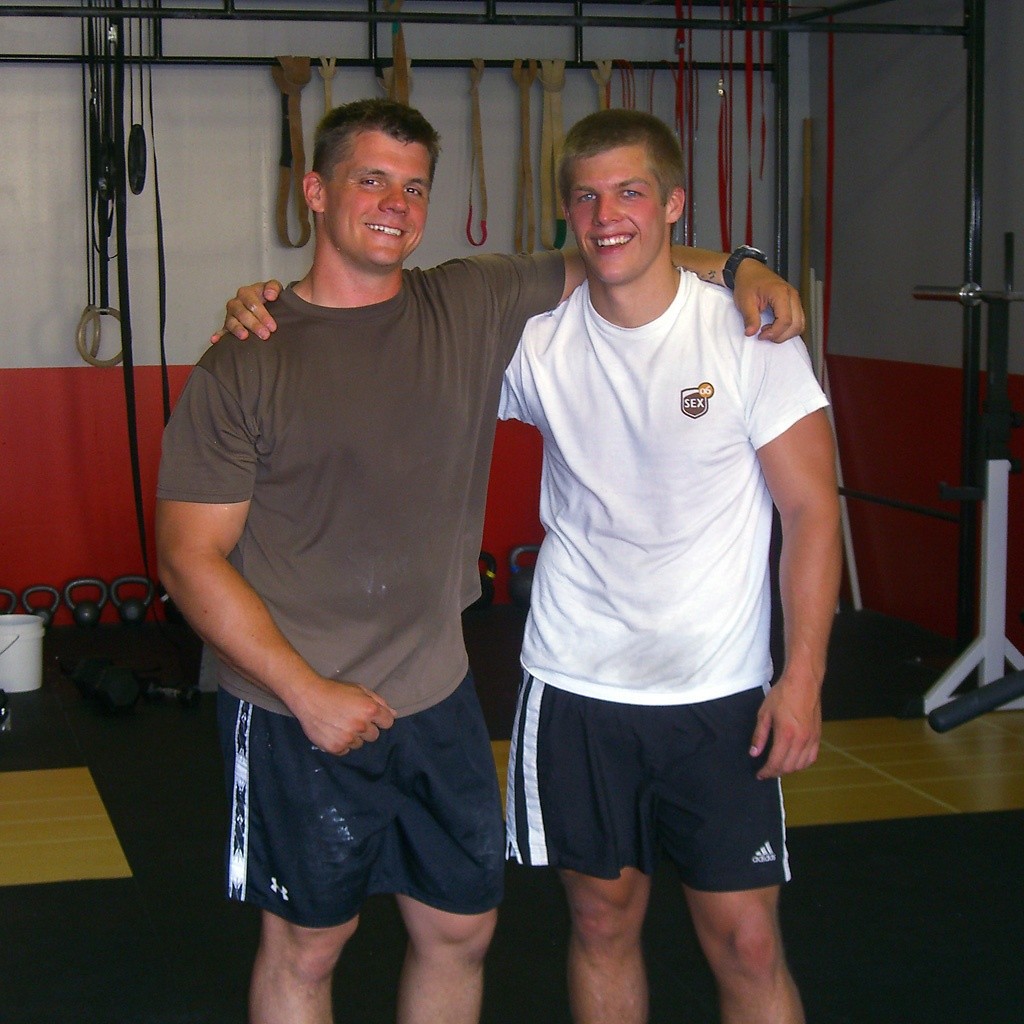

[0,615,45,694]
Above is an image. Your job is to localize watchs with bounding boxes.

[722,245,769,288]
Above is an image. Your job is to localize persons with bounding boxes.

[210,111,843,1023]
[160,94,806,1023]
